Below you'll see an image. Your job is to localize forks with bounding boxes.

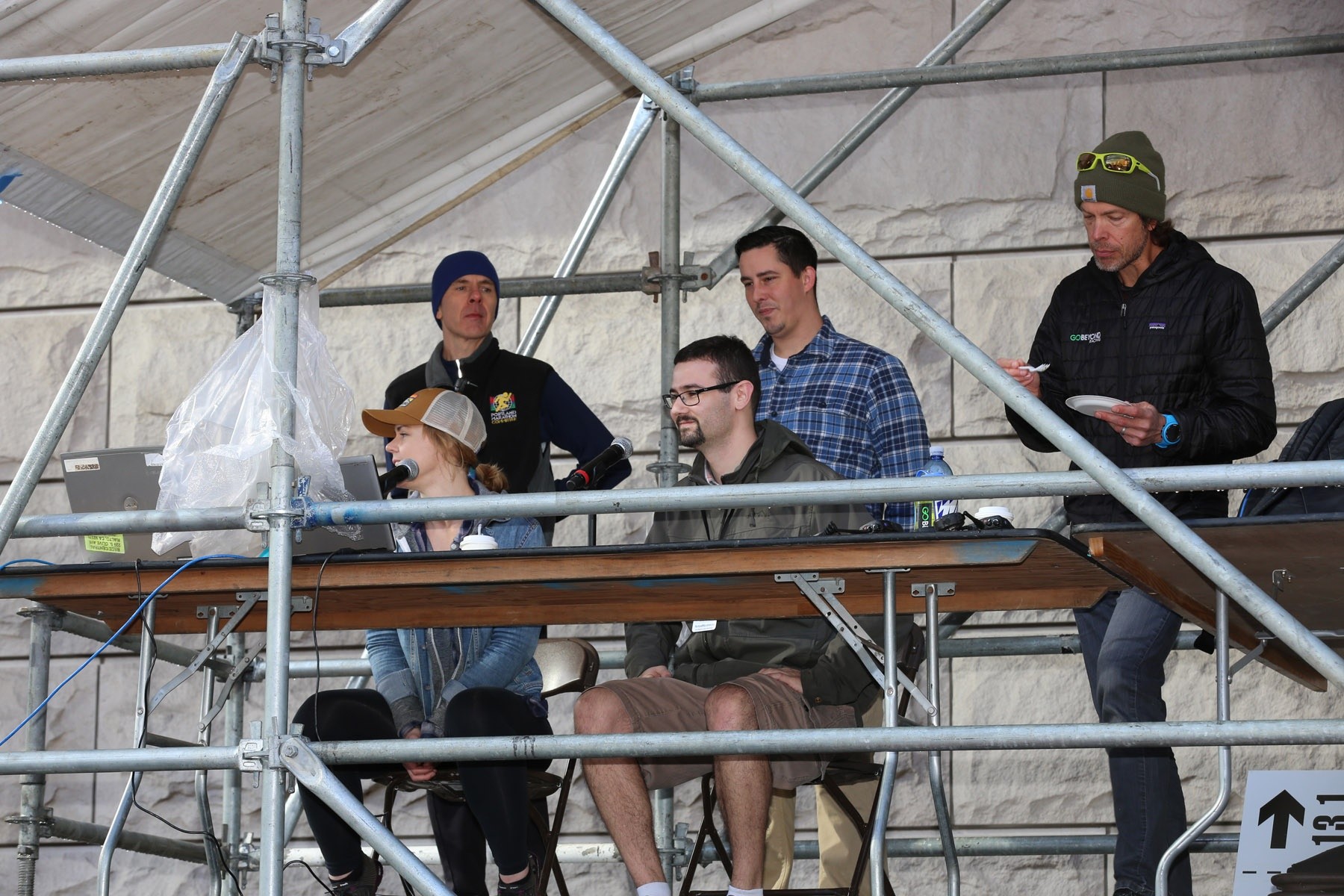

[1018,363,1051,373]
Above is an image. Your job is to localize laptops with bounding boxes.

[292,454,397,557]
[59,445,199,563]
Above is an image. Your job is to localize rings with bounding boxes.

[1121,427,1125,435]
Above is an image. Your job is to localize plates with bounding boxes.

[1066,395,1135,420]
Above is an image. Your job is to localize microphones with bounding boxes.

[379,457,419,494]
[566,436,633,490]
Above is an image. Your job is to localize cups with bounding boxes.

[974,506,1014,525]
[459,534,498,551]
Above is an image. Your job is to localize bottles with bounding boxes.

[913,446,959,531]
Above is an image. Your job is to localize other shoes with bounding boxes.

[324,855,383,896]
[497,851,545,896]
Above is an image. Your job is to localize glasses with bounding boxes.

[662,381,741,409]
[1075,150,1161,192]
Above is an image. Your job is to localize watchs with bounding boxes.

[1154,414,1182,448]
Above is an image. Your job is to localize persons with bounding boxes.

[572,333,909,896]
[384,251,630,896]
[994,129,1277,896]
[292,386,556,896]
[734,225,934,896]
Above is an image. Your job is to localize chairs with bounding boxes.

[369,638,600,896]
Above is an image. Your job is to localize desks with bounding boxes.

[678,622,925,896]
[0,528,1131,896]
[1069,513,1344,896]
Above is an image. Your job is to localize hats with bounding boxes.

[1075,131,1166,222]
[432,251,499,331]
[361,387,488,457]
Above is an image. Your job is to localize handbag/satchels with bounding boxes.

[152,271,364,562]
